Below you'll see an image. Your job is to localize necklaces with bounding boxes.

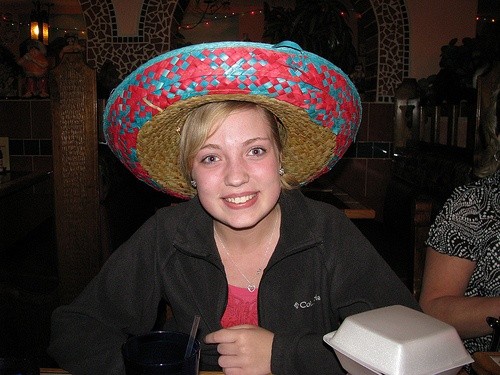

[215,210,278,293]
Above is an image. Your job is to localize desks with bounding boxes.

[0,171,55,249]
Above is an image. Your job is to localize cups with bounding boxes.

[121,329,201,375]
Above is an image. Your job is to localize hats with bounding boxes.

[103,40,363,200]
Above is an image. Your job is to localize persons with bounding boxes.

[19,33,86,97]
[418,90,500,375]
[48,40,425,375]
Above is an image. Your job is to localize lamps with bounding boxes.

[26,0,56,46]
